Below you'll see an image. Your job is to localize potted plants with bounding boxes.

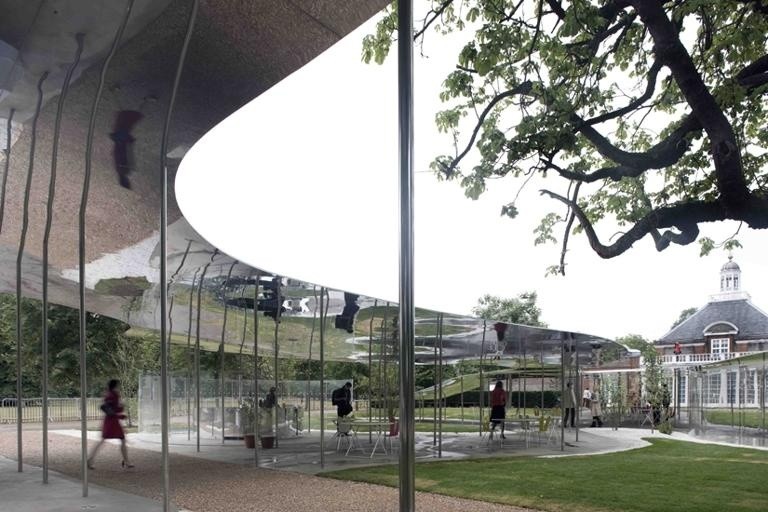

[237,401,279,450]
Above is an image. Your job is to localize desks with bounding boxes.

[339,419,394,458]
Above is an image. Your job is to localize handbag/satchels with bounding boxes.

[100,401,116,417]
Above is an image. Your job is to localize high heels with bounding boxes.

[122,460,136,467]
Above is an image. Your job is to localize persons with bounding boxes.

[556,383,671,427]
[335,381,353,438]
[87,379,136,469]
[562,332,577,366]
[564,381,577,427]
[343,292,360,333]
[488,381,507,440]
[489,323,513,360]
[108,83,159,190]
[672,342,681,361]
[263,386,280,433]
[590,341,603,367]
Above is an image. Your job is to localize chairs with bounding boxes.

[380,420,403,455]
[476,405,562,449]
[327,419,355,452]
